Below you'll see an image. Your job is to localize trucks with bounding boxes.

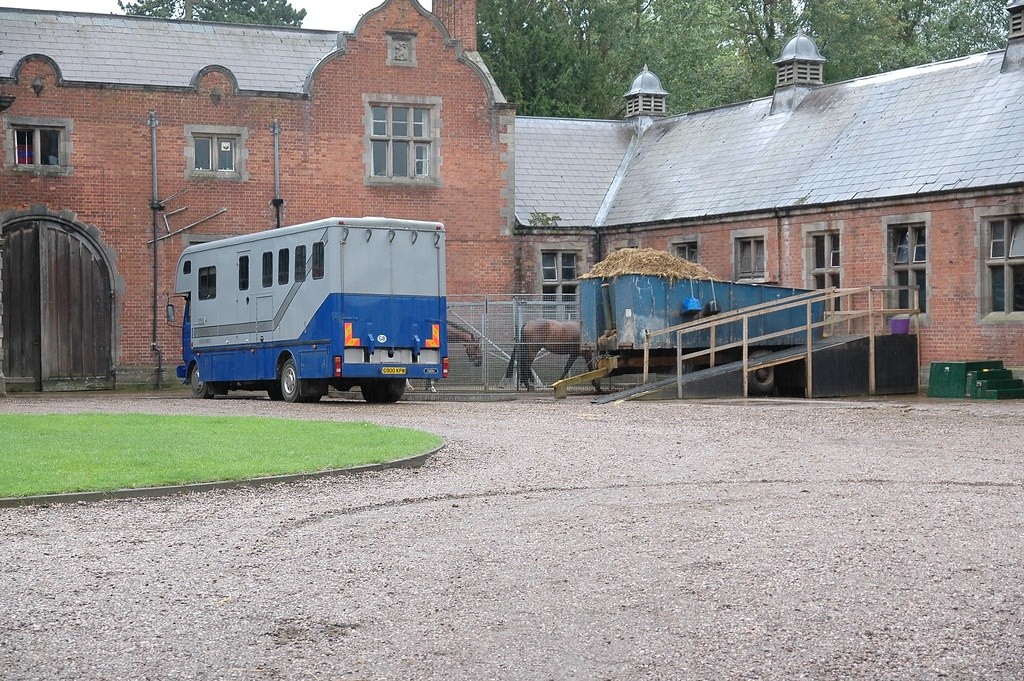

[165,215,449,404]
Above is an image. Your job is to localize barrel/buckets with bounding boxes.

[890,319,910,334]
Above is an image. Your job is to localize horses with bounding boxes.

[405,319,483,393]
[518,318,605,395]
